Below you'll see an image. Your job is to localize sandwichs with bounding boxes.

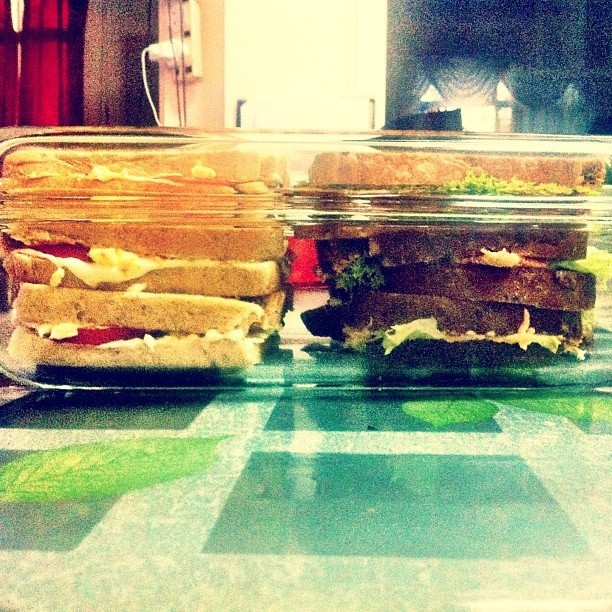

[301,152,609,361]
[2,129,288,363]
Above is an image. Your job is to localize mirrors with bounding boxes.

[383,1,611,138]
